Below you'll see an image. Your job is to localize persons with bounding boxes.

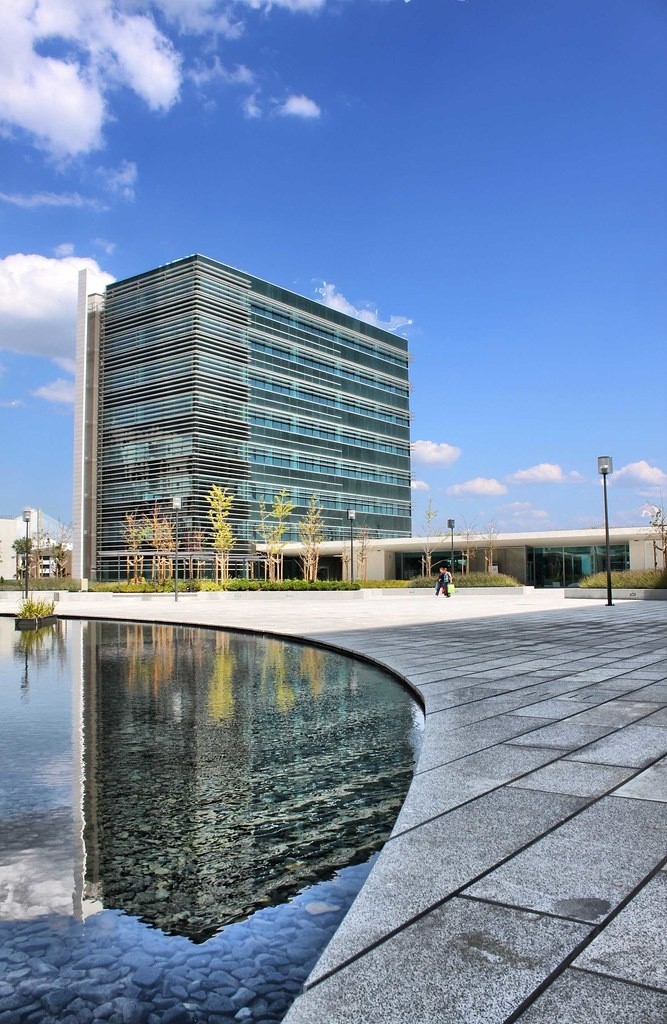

[433,567,453,599]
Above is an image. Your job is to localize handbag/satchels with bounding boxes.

[442,587,446,594]
[447,583,455,594]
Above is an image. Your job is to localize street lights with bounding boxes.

[346,508,356,582]
[172,497,182,602]
[599,455,613,606]
[23,509,32,599]
[447,518,455,578]
